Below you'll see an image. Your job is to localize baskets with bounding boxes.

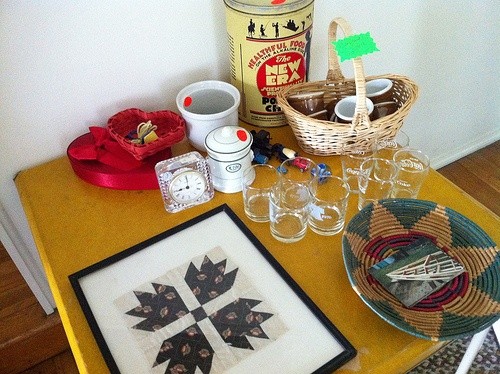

[276,18,419,157]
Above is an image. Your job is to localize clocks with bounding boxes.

[155,153,215,214]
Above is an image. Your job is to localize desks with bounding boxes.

[14,119,500,374]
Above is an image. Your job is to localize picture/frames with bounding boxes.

[69,203,356,374]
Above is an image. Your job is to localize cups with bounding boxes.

[341,127,430,211]
[205,125,256,195]
[289,77,398,125]
[175,79,240,152]
[242,156,350,243]
